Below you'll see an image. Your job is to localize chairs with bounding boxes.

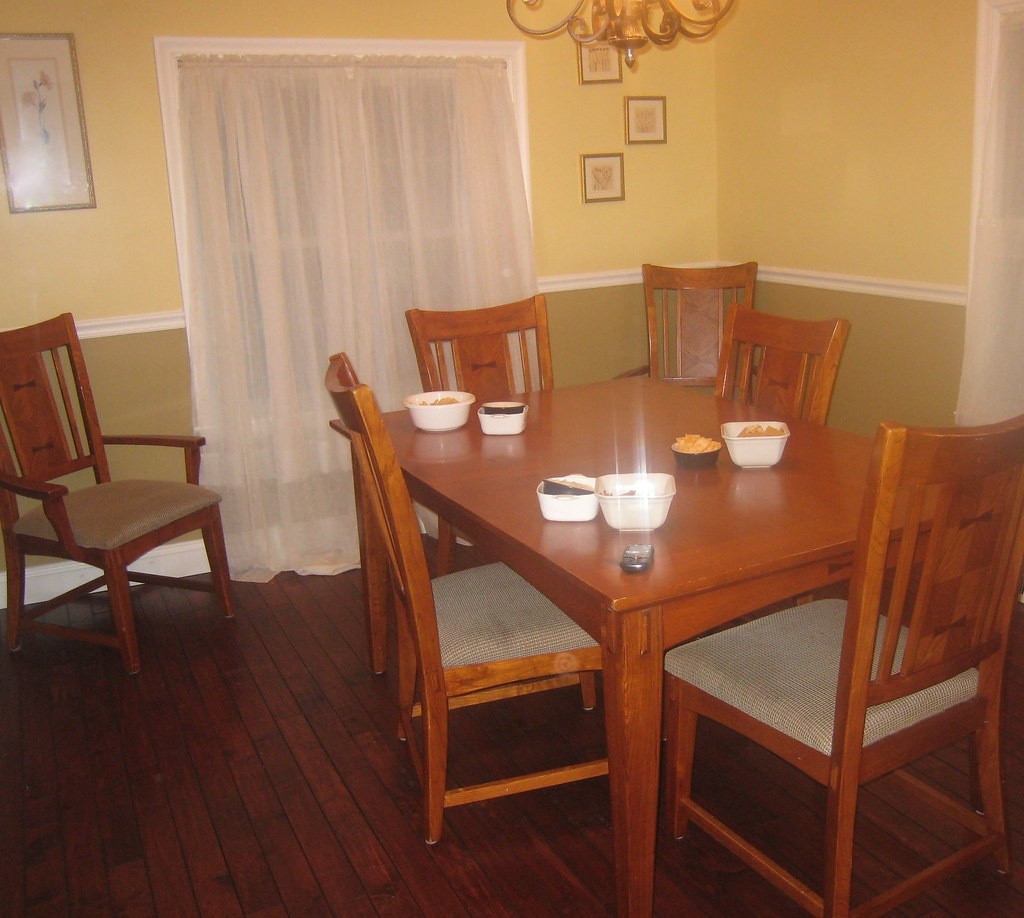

[612,262,758,386]
[0,313,235,674]
[715,302,849,425]
[406,294,554,576]
[325,352,609,844]
[665,415,1024,918]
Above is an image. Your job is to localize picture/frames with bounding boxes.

[579,35,624,84]
[626,95,667,143]
[0,32,96,214]
[581,153,624,203]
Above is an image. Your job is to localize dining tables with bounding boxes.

[329,377,941,918]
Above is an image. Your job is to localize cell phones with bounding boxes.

[620,543,654,573]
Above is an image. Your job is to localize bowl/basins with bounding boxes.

[597,472,678,535]
[538,471,599,522]
[483,399,524,416]
[544,476,592,500]
[478,406,530,437]
[719,419,791,470]
[672,437,721,468]
[402,389,474,433]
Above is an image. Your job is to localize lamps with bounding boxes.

[506,0,733,69]
